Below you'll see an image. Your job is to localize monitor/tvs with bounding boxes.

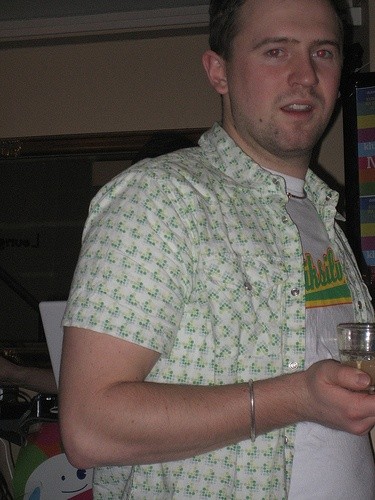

[342,71,375,286]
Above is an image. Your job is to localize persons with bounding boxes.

[60,0,375,500]
[1,358,58,394]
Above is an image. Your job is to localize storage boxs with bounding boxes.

[0,158,134,343]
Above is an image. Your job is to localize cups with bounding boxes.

[335,322,375,394]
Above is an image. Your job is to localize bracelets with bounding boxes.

[249,378,255,440]
